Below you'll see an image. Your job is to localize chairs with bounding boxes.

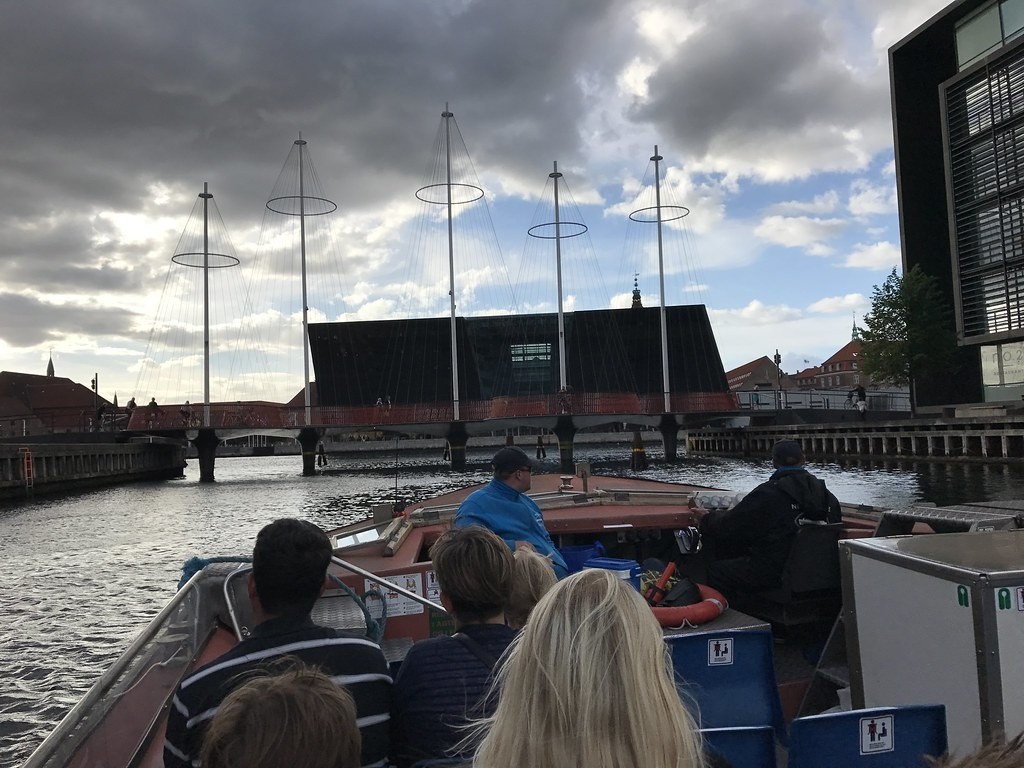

[787,705,950,768]
[663,630,789,768]
[751,521,846,644]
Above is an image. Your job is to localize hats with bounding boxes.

[774,438,802,465]
[491,446,539,469]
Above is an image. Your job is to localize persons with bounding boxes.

[692,439,842,645]
[97,397,194,428]
[453,446,568,581]
[849,384,868,420]
[376,395,392,418]
[556,383,575,415]
[162,518,393,768]
[394,528,729,768]
[753,385,760,410]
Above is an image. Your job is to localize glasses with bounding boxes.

[513,466,531,472]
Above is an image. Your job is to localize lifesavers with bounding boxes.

[645,581,728,625]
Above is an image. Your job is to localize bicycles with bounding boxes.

[844,391,864,411]
[171,411,203,430]
